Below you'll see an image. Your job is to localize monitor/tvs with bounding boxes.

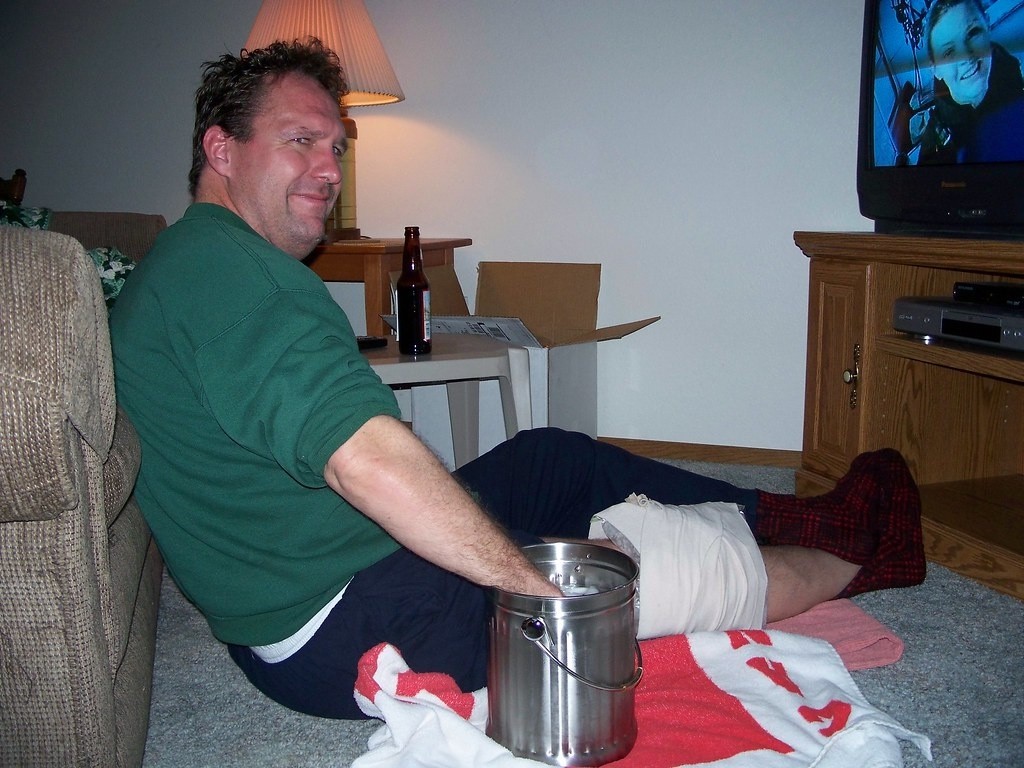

[856,0,1024,242]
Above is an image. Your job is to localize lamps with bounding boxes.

[239,0,405,243]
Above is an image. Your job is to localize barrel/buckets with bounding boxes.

[486,541,644,768]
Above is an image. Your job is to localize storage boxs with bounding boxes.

[379,260,661,475]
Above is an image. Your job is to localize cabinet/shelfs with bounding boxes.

[793,231,1024,602]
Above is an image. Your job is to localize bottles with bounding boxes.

[396,226,432,355]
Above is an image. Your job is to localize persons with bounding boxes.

[108,34,927,718]
[917,0,1024,165]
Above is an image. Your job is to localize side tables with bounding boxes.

[298,238,469,336]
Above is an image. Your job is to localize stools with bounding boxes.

[361,333,534,469]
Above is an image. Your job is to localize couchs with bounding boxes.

[0,167,167,768]
